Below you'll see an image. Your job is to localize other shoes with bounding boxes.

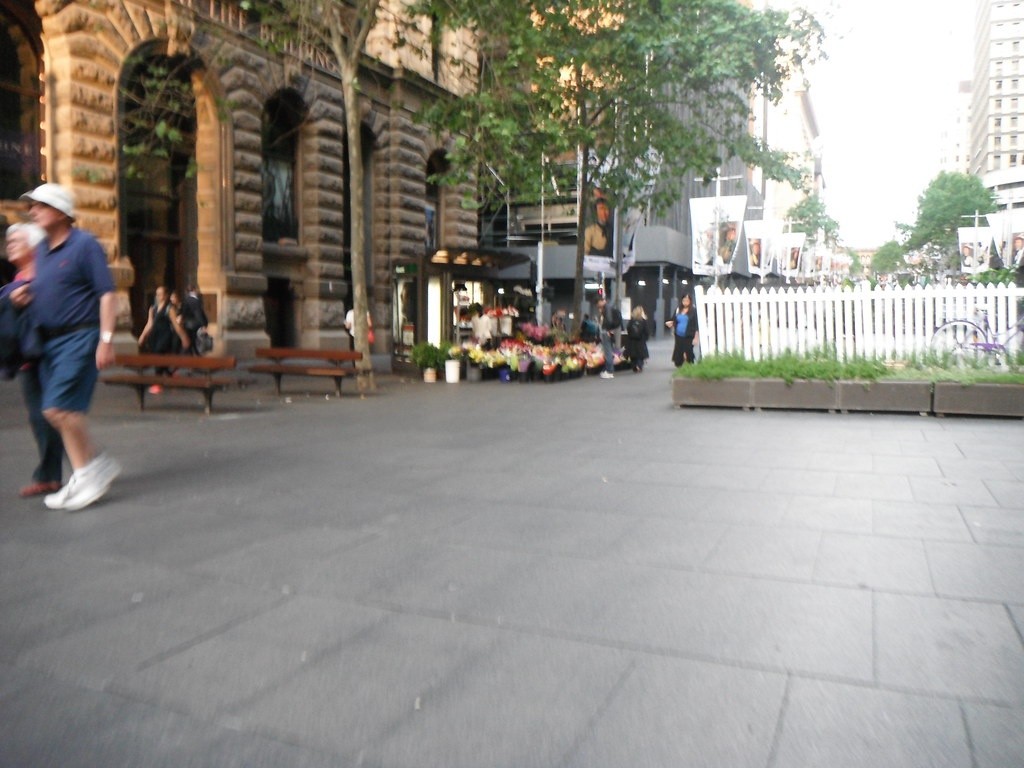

[19,482,60,497]
[148,385,164,396]
[44,453,122,512]
[600,370,614,378]
[172,373,183,396]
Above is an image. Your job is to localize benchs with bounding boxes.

[250,346,362,398]
[96,352,235,414]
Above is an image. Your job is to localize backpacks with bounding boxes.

[627,319,643,340]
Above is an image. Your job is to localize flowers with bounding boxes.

[450,302,625,375]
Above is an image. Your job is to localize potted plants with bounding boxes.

[672,346,1024,418]
[438,340,461,383]
[411,340,441,383]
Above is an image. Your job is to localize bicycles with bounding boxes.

[930,304,1022,378]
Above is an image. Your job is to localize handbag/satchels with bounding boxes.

[197,327,213,353]
[368,327,375,345]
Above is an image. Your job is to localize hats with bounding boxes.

[19,184,80,224]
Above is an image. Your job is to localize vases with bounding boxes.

[466,361,631,383]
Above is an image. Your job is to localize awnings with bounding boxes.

[427,244,530,269]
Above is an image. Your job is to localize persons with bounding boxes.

[344,309,372,367]
[622,306,650,373]
[137,286,210,396]
[18,182,123,510]
[664,295,698,366]
[584,196,611,256]
[598,299,614,378]
[1013,232,1023,267]
[551,311,589,335]
[0,222,61,497]
